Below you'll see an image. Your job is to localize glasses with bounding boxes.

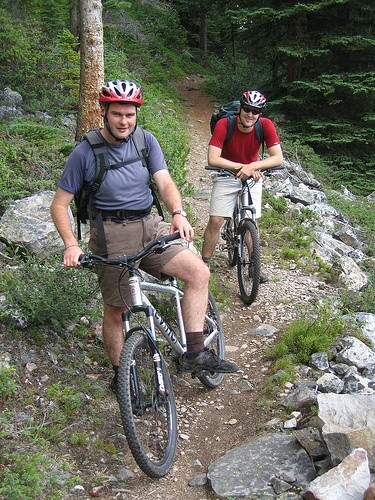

[241,107,260,115]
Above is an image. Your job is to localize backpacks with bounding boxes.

[210,101,265,160]
[74,127,165,240]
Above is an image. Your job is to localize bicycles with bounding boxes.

[68,230,226,480]
[204,163,286,308]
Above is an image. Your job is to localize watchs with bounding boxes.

[172,210,187,219]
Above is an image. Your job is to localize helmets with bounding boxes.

[240,91,267,109]
[99,79,144,106]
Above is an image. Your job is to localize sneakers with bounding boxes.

[206,263,210,270]
[110,376,145,416]
[249,270,270,283]
[179,345,240,373]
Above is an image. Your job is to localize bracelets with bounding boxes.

[65,244,78,250]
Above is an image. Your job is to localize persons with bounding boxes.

[201,91,283,283]
[50,79,238,404]
